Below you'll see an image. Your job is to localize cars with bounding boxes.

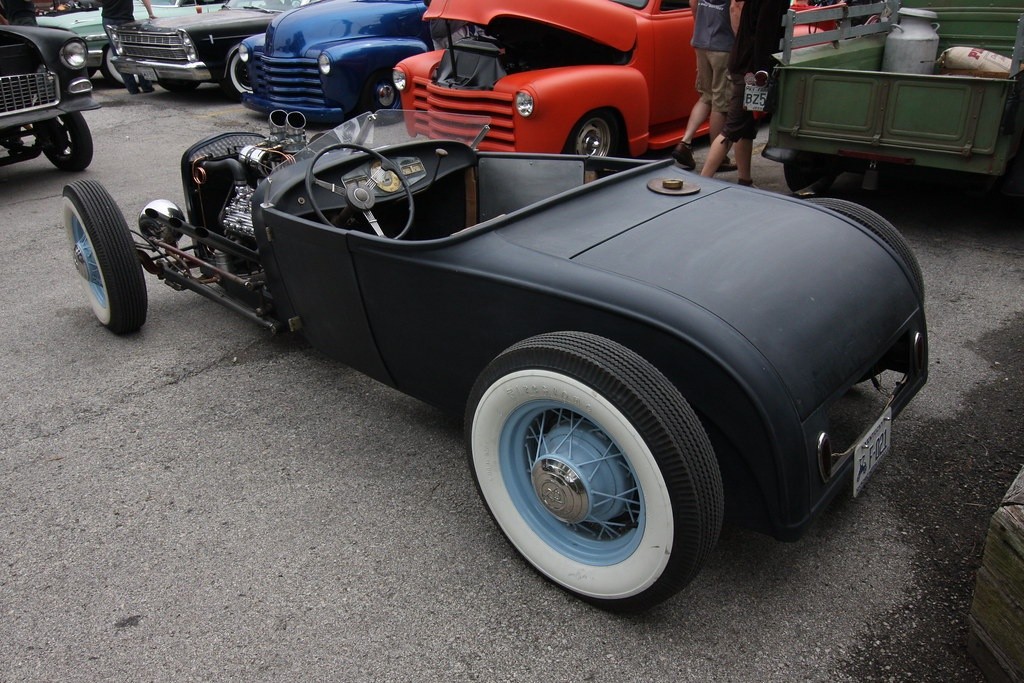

[0,0,102,173]
[104,0,320,103]
[62,109,934,614]
[239,0,430,126]
[35,0,303,90]
[391,0,839,158]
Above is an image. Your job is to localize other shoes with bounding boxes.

[738,178,758,189]
[144,87,155,92]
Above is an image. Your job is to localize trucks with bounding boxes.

[760,0,1024,219]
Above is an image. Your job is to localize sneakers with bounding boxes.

[671,141,696,170]
[717,156,737,172]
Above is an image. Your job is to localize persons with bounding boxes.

[671,0,737,172]
[0,0,38,26]
[808,0,885,38]
[700,0,791,190]
[101,0,158,95]
